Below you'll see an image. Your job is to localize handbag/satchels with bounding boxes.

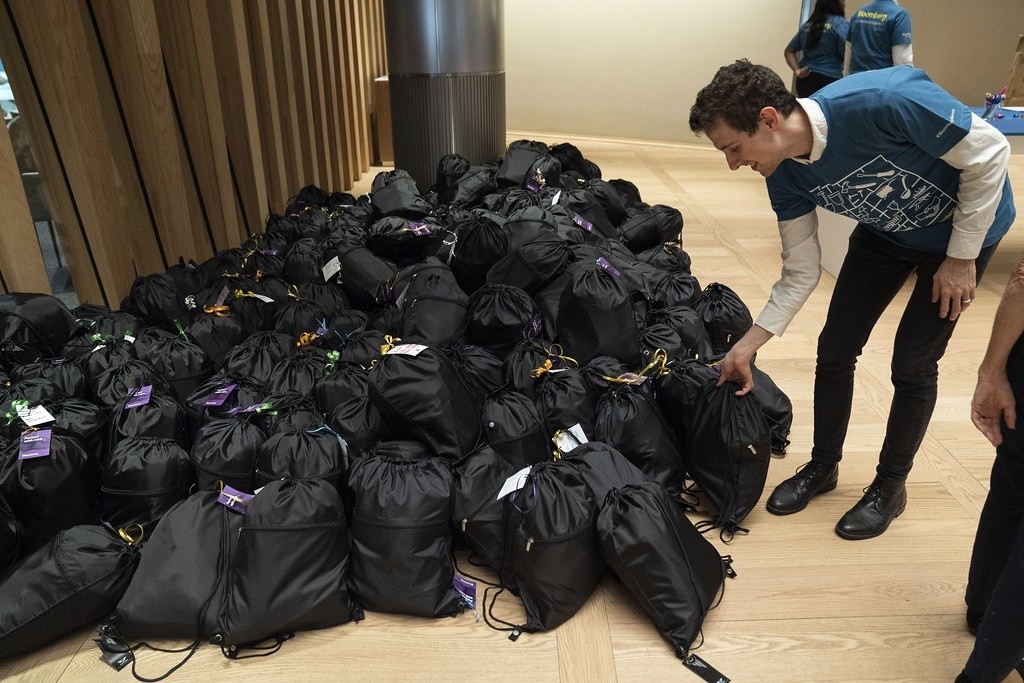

[0,138,796,683]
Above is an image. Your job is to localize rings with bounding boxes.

[962,299,970,303]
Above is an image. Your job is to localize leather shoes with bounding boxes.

[765,458,840,516]
[834,472,908,541]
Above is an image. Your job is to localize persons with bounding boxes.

[688,58,1016,540]
[849,0,914,75]
[784,0,850,98]
[953,256,1024,683]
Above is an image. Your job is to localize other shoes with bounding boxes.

[965,607,985,637]
